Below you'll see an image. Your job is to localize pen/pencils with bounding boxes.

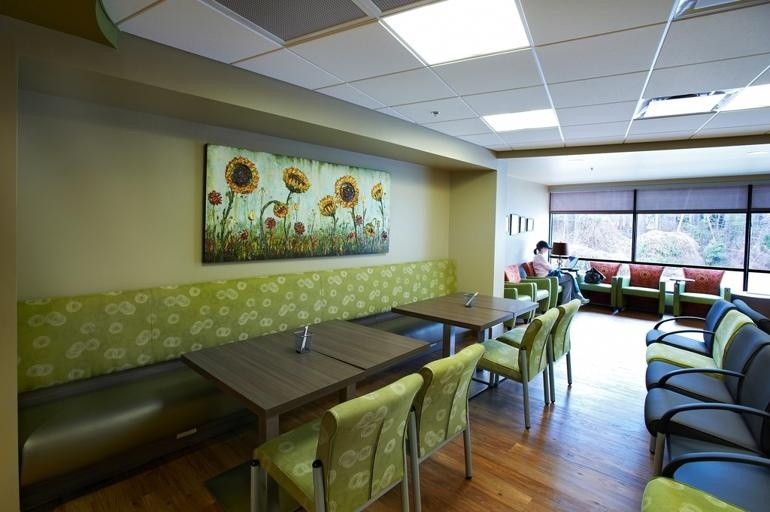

[465,292,479,306]
[301,325,308,351]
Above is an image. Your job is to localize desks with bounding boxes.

[182,321,432,512]
[560,268,579,276]
[392,292,539,400]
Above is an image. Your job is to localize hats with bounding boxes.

[537,241,553,250]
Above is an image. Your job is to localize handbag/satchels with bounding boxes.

[584,269,601,284]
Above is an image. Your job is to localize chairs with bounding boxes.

[253,374,424,512]
[505,298,581,403]
[411,342,485,512]
[643,300,770,512]
[673,267,731,317]
[616,264,665,315]
[577,262,621,308]
[466,308,559,429]
[504,261,573,311]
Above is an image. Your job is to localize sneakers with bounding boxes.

[579,297,591,304]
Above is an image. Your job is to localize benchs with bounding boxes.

[18,310,473,491]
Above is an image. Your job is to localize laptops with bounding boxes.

[560,256,579,270]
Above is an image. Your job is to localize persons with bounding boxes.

[533,240,590,306]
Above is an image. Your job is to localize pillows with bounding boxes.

[683,267,724,295]
[629,264,665,289]
[590,261,622,284]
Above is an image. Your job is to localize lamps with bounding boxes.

[551,241,568,268]
